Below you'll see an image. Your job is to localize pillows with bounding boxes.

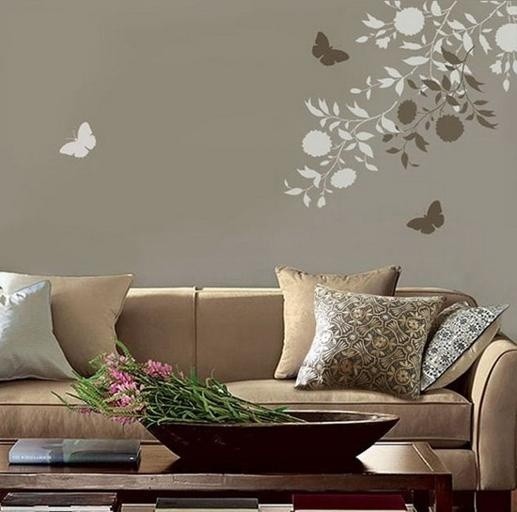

[274,264,402,379]
[0,273,134,377]
[0,280,82,382]
[293,283,447,400]
[421,302,511,392]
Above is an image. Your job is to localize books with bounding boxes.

[1,491,416,512]
[8,438,141,463]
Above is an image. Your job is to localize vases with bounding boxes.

[136,410,400,463]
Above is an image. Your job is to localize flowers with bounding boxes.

[51,340,307,429]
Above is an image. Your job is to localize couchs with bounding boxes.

[0,288,517,512]
